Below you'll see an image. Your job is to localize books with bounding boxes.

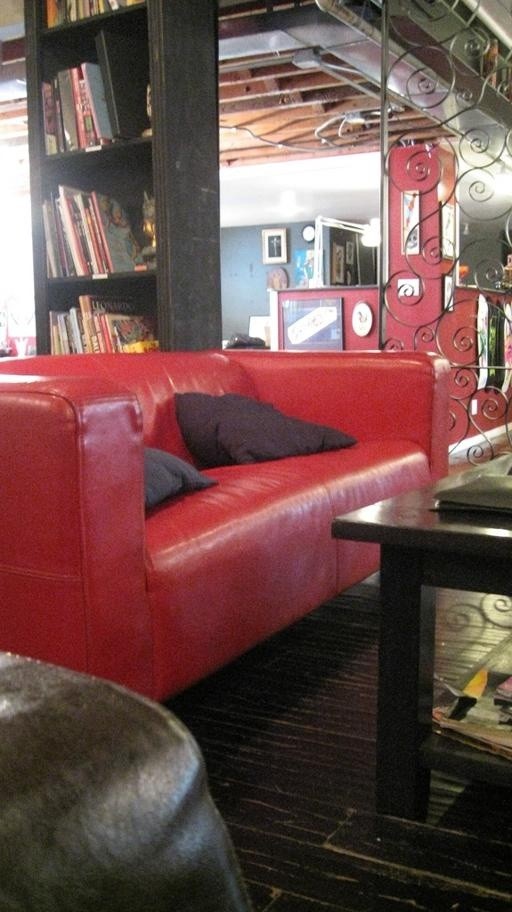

[46,1,157,354]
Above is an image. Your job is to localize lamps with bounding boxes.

[308,216,381,289]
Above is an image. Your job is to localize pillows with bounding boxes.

[143,441,222,525]
[173,390,357,473]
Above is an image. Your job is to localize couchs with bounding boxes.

[0,351,450,709]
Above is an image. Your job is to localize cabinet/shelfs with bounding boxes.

[23,0,224,352]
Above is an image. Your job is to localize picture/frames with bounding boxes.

[441,273,457,313]
[351,301,375,339]
[331,239,347,285]
[246,314,272,350]
[281,299,346,354]
[261,227,289,266]
[400,189,423,256]
[438,199,457,262]
[302,224,316,242]
